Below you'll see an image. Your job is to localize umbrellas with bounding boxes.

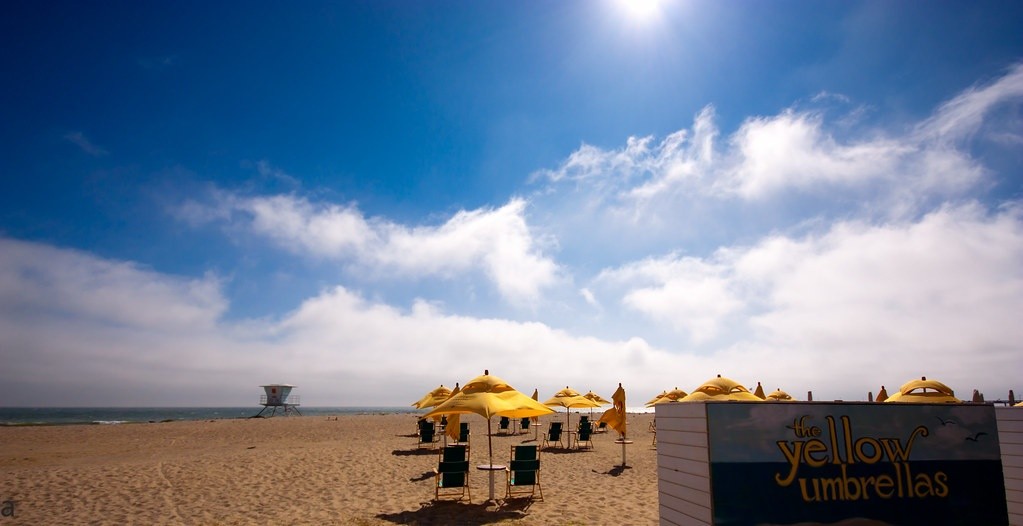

[644,390,668,405]
[419,369,559,468]
[876,386,888,403]
[543,386,601,448]
[582,390,612,421]
[411,385,453,441]
[445,384,460,444]
[765,388,796,401]
[754,382,766,400]
[595,383,626,439]
[646,386,689,407]
[882,375,961,405]
[679,374,765,401]
[530,388,538,438]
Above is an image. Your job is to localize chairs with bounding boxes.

[542,422,565,448]
[497,416,510,435]
[518,417,531,435]
[415,416,472,505]
[594,422,607,433]
[571,416,594,449]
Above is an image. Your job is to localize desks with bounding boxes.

[448,443,466,446]
[531,424,542,438]
[476,465,506,499]
[615,440,633,469]
[438,431,449,448]
[430,419,438,429]
[510,420,519,433]
[563,430,576,450]
[588,419,597,434]
[435,424,445,440]
